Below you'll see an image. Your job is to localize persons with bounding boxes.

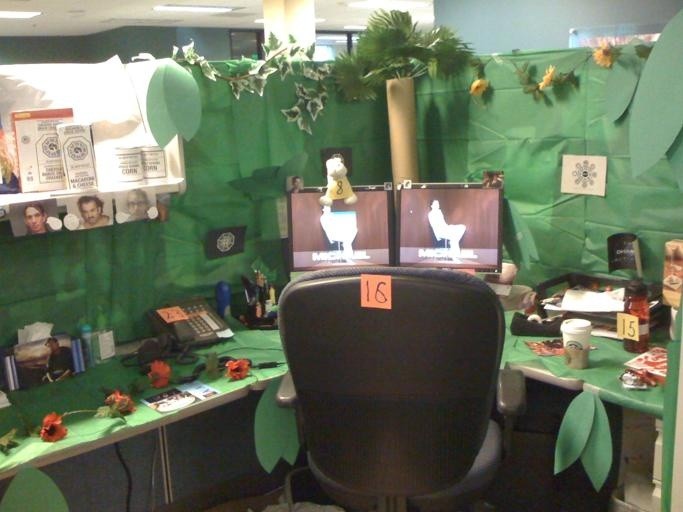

[321,203,357,257]
[24,204,49,236]
[288,177,303,194]
[77,195,111,229]
[125,189,152,222]
[427,199,466,255]
[41,339,75,382]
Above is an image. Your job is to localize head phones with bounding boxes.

[120,332,173,371]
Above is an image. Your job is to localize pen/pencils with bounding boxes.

[242,269,276,319]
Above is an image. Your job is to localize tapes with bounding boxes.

[526,313,563,324]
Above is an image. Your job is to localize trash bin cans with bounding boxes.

[611,482,655,512]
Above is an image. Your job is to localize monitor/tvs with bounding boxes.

[395,182,504,273]
[288,185,395,272]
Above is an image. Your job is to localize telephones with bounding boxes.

[144,296,235,351]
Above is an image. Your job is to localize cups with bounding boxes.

[561,318,592,369]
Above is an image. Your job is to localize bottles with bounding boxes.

[623,283,649,352]
[215,279,231,319]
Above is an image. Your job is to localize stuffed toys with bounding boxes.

[319,157,358,206]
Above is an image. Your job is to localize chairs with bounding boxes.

[276,265,527,511]
